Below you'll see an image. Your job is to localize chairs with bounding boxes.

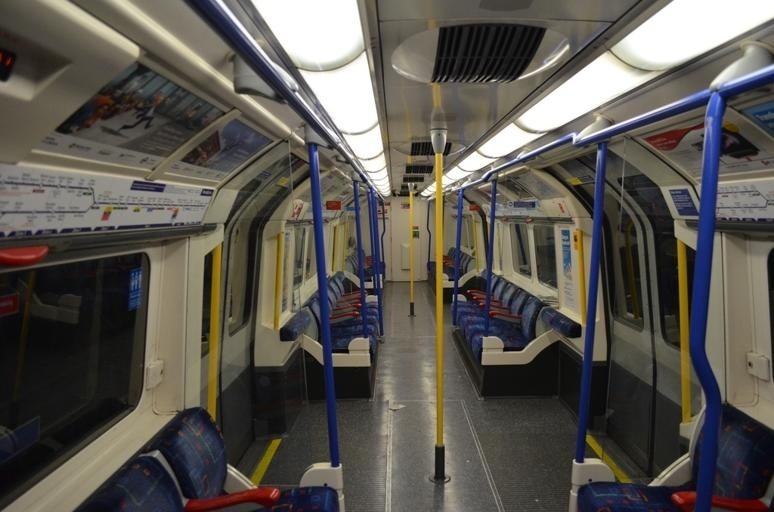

[72,452,279,512]
[576,403,774,512]
[280,271,383,399]
[427,247,475,281]
[141,405,341,512]
[452,269,583,397]
[345,245,386,280]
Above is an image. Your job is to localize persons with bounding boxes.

[61,92,164,147]
